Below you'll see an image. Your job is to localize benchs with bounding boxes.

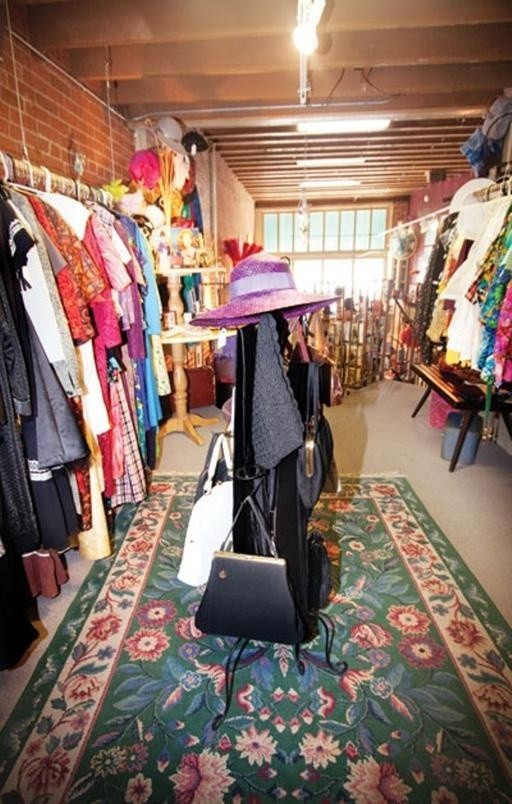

[409,360,512,472]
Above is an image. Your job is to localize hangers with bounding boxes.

[1,150,40,199]
[485,182,497,203]
[41,166,54,201]
[70,174,82,207]
[84,179,118,215]
[507,176,512,203]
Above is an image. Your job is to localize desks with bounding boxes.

[154,328,240,447]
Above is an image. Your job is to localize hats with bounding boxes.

[189,255,340,326]
[448,179,498,213]
[154,117,186,155]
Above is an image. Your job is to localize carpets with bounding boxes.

[1,472,512,804]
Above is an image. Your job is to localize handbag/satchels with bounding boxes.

[175,435,233,588]
[192,433,230,504]
[295,363,333,510]
[308,532,331,610]
[194,496,299,644]
